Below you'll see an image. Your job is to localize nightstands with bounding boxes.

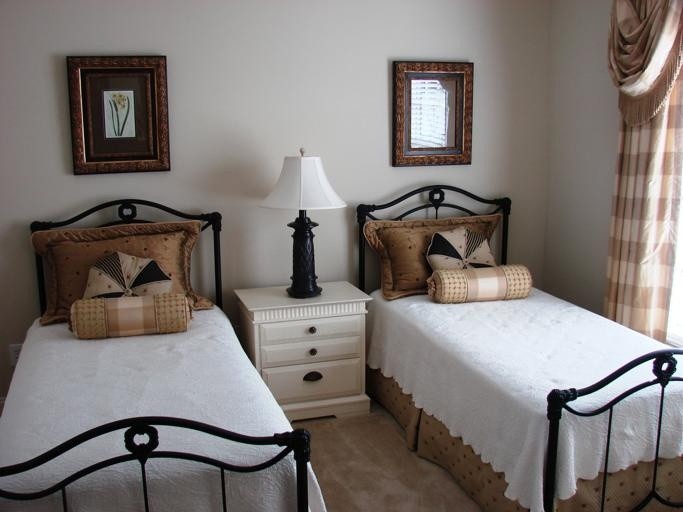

[232,280,372,424]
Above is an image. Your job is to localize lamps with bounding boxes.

[258,147,347,300]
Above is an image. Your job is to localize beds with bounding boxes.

[0,200,328,512]
[356,185,682,512]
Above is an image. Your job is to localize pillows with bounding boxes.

[363,212,502,301]
[426,265,532,304]
[70,295,191,340]
[82,251,173,302]
[426,224,498,271]
[30,220,214,326]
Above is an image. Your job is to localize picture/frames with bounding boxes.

[65,54,171,176]
[391,61,474,167]
